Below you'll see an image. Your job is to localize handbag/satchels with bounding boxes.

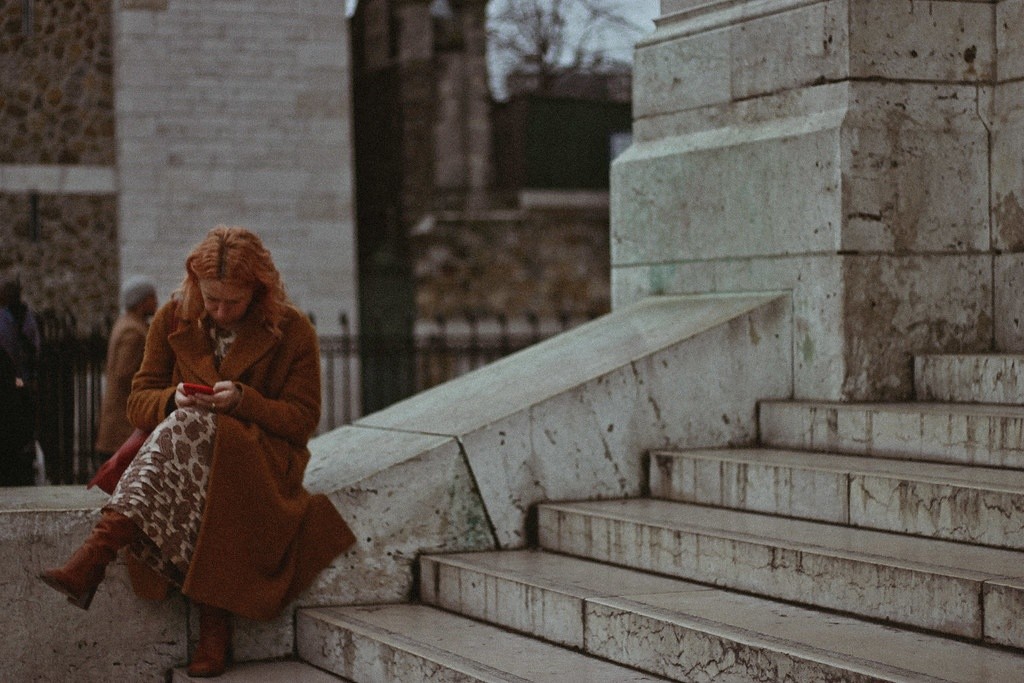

[90,427,149,495]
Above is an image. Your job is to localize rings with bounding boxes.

[211,402,216,410]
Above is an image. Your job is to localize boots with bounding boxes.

[42,508,141,610]
[186,604,235,678]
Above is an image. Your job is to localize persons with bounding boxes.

[94,275,160,463]
[35,223,356,677]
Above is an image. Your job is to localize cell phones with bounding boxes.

[183,383,214,397]
